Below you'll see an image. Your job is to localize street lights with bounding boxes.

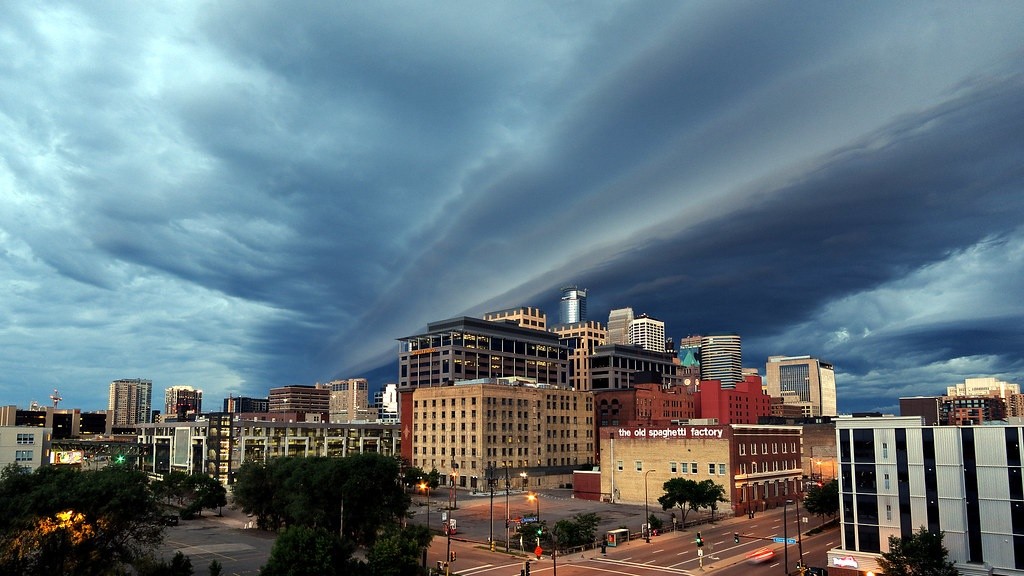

[644,469,656,543]
[809,455,823,489]
[784,499,794,575]
[452,470,457,508]
[746,462,756,520]
[420,484,429,532]
[525,494,543,560]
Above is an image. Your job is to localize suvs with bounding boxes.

[158,516,179,527]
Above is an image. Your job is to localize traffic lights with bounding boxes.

[696,531,704,548]
[535,523,542,538]
[734,532,741,544]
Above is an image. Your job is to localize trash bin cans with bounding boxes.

[437,560,444,571]
[566,483,572,489]
[601,545,606,553]
